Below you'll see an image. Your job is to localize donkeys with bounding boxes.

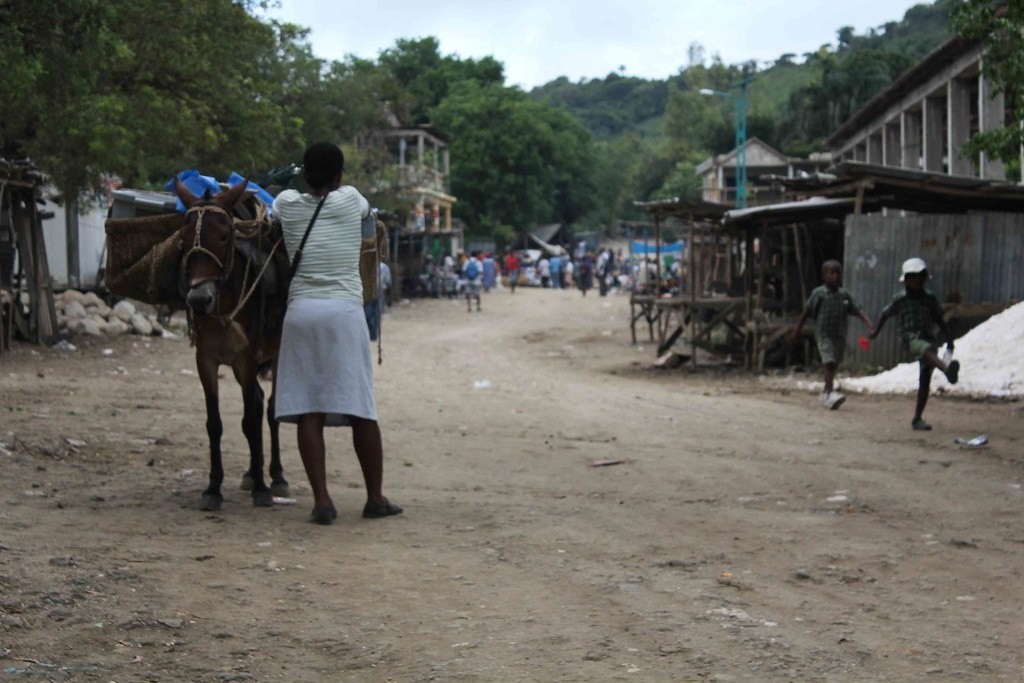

[174,175,292,511]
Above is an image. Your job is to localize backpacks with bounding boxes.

[466,262,479,279]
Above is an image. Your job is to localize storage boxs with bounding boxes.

[109,188,177,217]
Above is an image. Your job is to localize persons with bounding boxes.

[271,141,404,525]
[789,260,875,410]
[868,257,960,430]
[363,243,688,342]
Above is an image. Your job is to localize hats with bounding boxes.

[304,142,347,173]
[899,258,933,282]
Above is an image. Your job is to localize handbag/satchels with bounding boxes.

[269,273,295,319]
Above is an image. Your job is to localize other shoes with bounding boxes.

[362,495,402,519]
[311,504,337,524]
[945,360,960,384]
[828,390,846,411]
[818,390,828,407]
[912,418,932,430]
